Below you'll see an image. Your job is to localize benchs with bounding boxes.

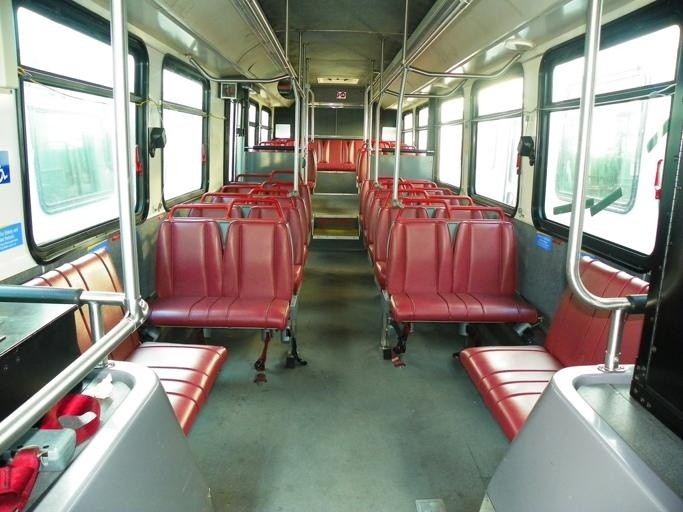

[459,255,650,441]
[21,249,227,436]
[318,138,396,171]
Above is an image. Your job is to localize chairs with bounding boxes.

[355,144,542,361]
[139,139,318,377]
